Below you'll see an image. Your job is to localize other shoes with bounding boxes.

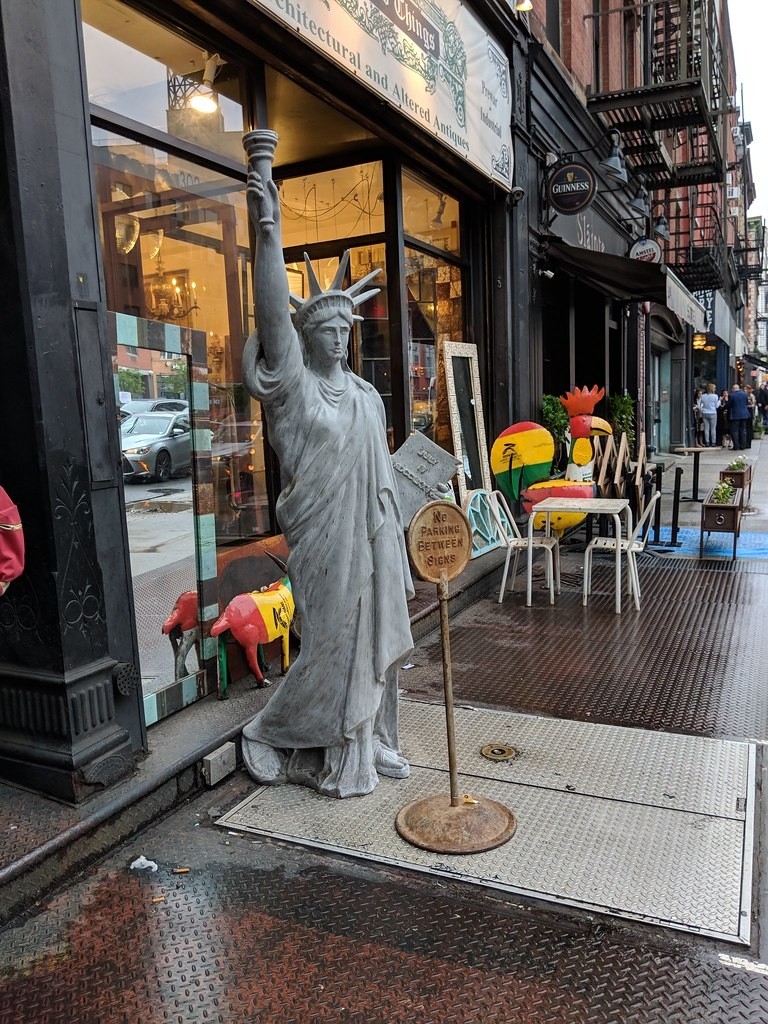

[723,443,732,448]
[696,443,705,447]
[705,442,716,446]
[729,448,745,451]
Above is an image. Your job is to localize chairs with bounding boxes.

[485,490,562,607]
[221,454,270,539]
[582,491,662,614]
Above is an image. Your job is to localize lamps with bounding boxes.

[145,228,201,323]
[605,152,633,185]
[616,203,668,239]
[96,185,142,255]
[556,128,623,174]
[632,189,653,218]
[189,51,229,114]
[597,175,646,212]
[429,194,449,232]
[635,221,670,242]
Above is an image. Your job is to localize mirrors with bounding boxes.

[407,341,436,446]
[442,341,504,560]
[104,312,221,729]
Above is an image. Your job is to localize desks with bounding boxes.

[528,497,643,614]
[674,446,722,503]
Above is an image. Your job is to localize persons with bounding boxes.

[743,385,756,440]
[239,132,413,798]
[694,389,706,448]
[757,381,768,434]
[720,389,733,448]
[699,384,724,447]
[727,384,751,450]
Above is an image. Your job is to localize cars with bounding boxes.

[122,412,189,482]
[120,399,189,412]
[210,419,265,480]
